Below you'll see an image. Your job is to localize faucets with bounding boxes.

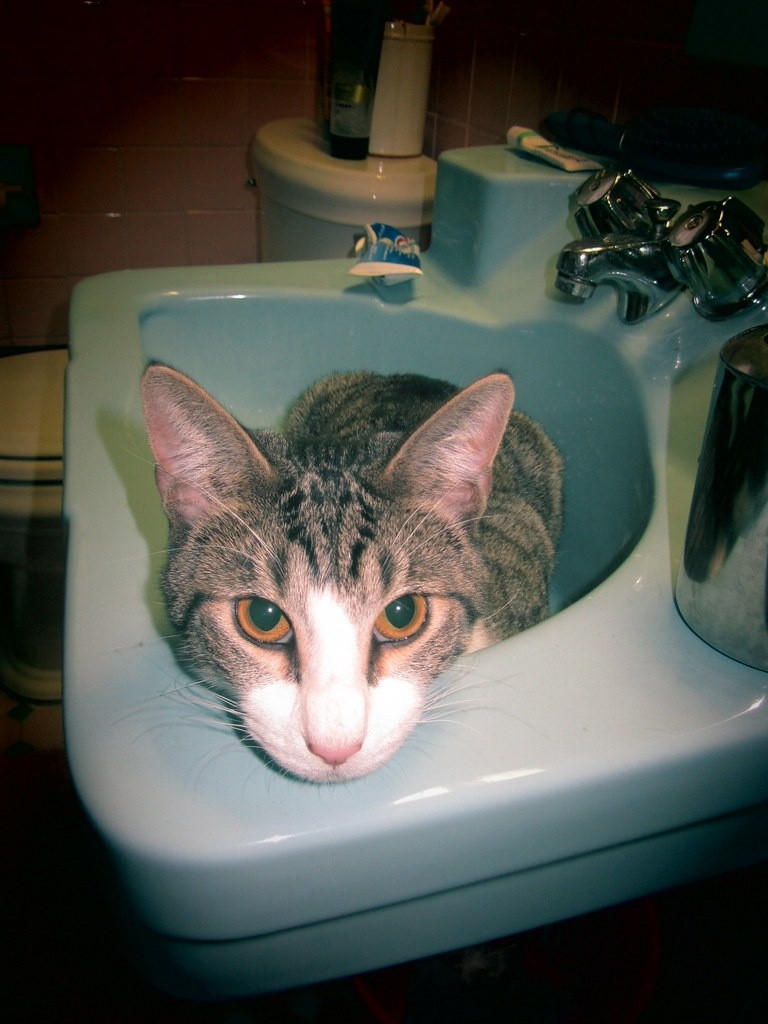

[553,161,685,332]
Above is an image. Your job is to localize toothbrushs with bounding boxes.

[423,0,436,21]
[427,2,450,28]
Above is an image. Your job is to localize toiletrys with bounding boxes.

[318,0,388,166]
[506,124,609,176]
[348,222,431,291]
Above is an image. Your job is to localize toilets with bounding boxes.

[2,120,443,722]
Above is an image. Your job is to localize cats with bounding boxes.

[138,359,565,786]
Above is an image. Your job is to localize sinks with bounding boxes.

[52,142,767,999]
